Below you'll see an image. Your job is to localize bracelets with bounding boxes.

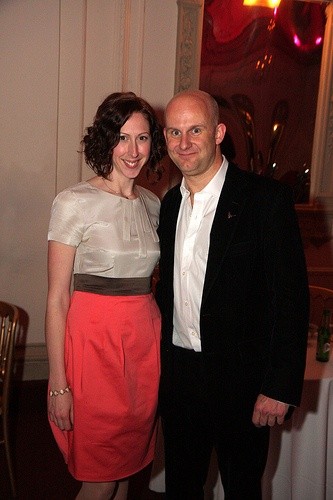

[49,385,70,397]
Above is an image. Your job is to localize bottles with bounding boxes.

[316,309,329,362]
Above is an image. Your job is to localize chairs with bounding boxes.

[0,299,29,500]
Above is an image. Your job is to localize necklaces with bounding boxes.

[101,177,134,197]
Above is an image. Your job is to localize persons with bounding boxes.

[46,91,164,500]
[156,88,310,500]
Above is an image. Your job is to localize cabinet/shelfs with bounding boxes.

[264,201,333,386]
